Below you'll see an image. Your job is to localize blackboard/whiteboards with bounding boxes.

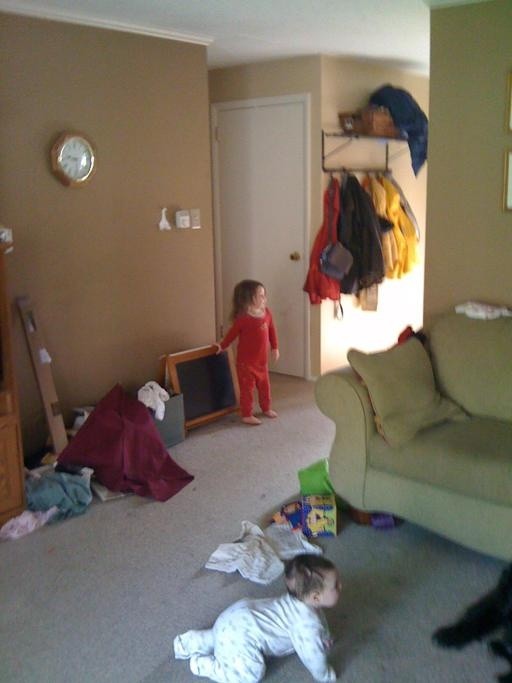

[168,344,241,428]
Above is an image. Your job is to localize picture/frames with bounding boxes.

[502,147,512,213]
[503,74,512,133]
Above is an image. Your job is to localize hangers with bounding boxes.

[329,166,380,186]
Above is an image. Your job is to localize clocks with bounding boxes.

[50,130,100,190]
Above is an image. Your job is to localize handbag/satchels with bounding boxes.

[319,180,355,281]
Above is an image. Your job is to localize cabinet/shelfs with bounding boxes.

[321,129,409,173]
[0,240,28,533]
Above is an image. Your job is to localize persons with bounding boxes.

[171,553,343,682]
[215,278,278,423]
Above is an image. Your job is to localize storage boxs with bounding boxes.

[338,111,402,138]
[148,393,186,449]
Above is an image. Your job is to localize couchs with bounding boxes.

[314,313,511,563]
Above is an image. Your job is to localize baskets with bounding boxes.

[336,106,398,138]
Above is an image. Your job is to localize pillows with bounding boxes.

[346,336,473,450]
